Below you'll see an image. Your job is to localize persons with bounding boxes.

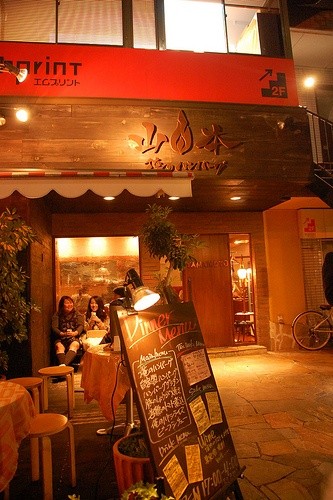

[82,296,110,331]
[112,287,132,301]
[52,295,83,366]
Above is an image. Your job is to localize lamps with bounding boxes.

[277,117,295,129]
[237,253,248,280]
[118,267,161,314]
[0,58,29,83]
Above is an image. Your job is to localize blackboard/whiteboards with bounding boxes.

[114,300,241,500]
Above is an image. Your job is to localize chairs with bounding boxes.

[7,377,42,411]
[38,364,78,418]
[9,413,76,500]
[236,322,257,343]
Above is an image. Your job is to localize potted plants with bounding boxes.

[114,202,208,492]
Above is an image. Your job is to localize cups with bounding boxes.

[67,329,72,338]
[91,312,96,317]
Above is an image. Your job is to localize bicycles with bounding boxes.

[291,305,333,350]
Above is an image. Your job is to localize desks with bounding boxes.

[0,379,35,500]
[234,312,255,343]
[80,341,143,437]
